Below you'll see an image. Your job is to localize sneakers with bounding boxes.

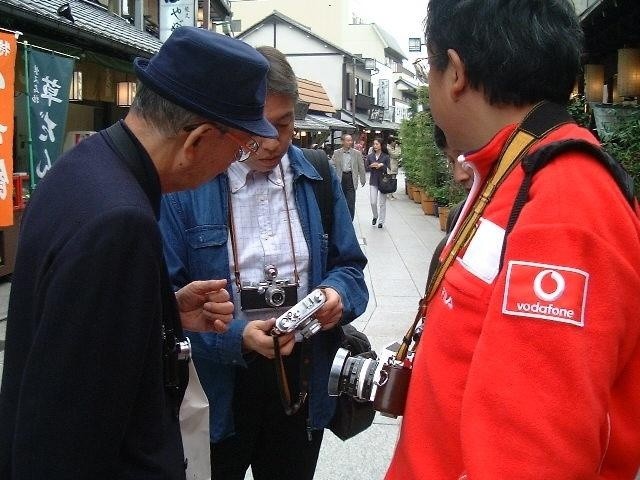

[372,218,382,228]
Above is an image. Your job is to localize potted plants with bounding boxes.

[398,84,468,230]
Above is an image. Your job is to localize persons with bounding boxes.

[0,25,277,479]
[381,0,640,480]
[433,124,475,194]
[156,46,369,479]
[329,134,402,228]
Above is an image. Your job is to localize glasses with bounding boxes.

[181,119,259,163]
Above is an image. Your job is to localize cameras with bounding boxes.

[160,329,192,390]
[270,288,327,339]
[327,347,412,420]
[240,264,298,311]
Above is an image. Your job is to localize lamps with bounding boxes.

[57,3,76,23]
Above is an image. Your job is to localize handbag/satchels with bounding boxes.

[377,174,397,194]
[323,325,375,443]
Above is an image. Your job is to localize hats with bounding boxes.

[132,25,279,139]
